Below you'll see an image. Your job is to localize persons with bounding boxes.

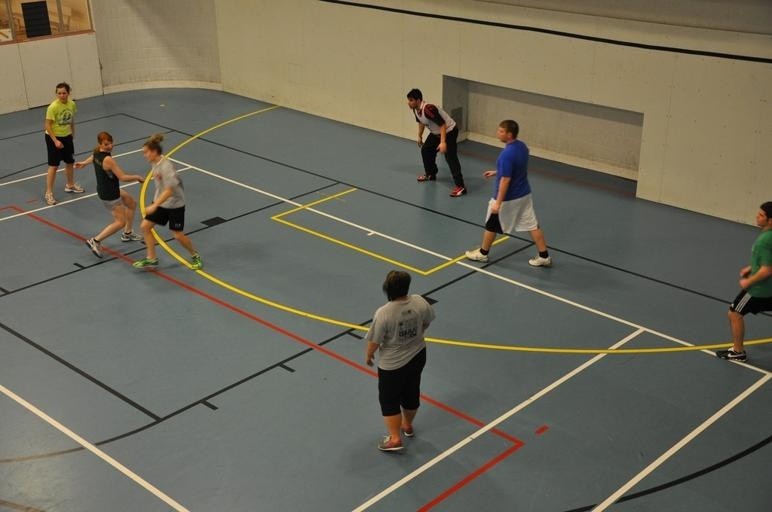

[712,201,771,360]
[406,88,466,197]
[133,134,204,271]
[73,131,144,259]
[466,119,550,267]
[44,80,86,205]
[364,270,436,452]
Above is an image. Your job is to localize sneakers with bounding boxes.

[716,350,747,363]
[404,425,414,437]
[417,175,436,181]
[466,249,488,262]
[379,436,402,450]
[450,186,466,196]
[45,184,202,270]
[529,256,551,266]
